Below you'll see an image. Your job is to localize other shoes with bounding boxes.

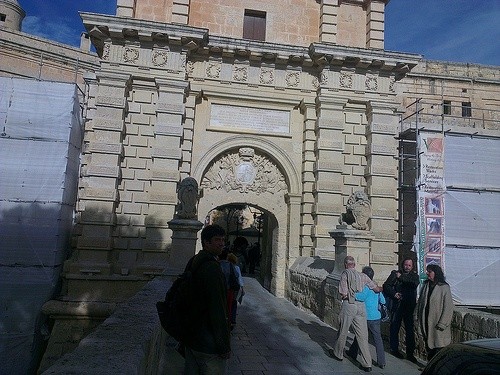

[391,351,404,359]
[407,354,417,363]
[328,349,343,361]
[359,365,372,372]
[343,349,355,360]
[376,364,386,369]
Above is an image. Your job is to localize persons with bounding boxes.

[219,241,260,331]
[417,265,453,364]
[343,267,386,369]
[182,224,231,375]
[382,258,420,362]
[328,256,372,372]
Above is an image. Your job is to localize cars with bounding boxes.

[420,338,500,375]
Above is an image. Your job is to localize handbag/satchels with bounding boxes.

[377,292,386,319]
[385,278,400,312]
[156,255,198,341]
